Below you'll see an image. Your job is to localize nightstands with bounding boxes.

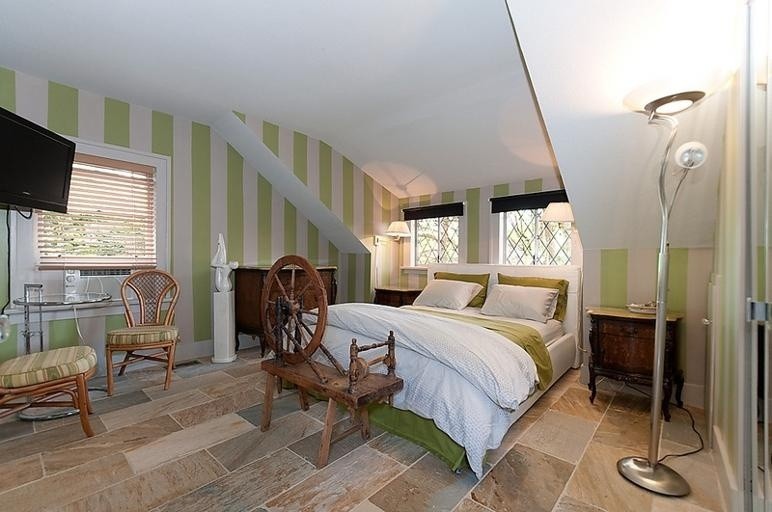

[585,303,687,422]
[373,284,424,306]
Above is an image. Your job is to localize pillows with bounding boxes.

[497,272,569,322]
[481,284,560,323]
[433,272,491,308]
[413,279,484,310]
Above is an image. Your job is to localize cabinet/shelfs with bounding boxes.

[234,264,338,359]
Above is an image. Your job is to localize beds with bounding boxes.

[264,263,582,476]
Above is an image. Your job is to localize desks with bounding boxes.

[13,284,113,423]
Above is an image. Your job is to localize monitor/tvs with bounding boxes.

[0,107,76,214]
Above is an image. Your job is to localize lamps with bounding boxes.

[614,89,710,501]
[536,201,573,231]
[374,221,414,247]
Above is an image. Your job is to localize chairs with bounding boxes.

[106,269,182,394]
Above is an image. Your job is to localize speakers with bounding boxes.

[64,270,80,293]
[64,293,80,301]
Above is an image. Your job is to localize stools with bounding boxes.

[0,344,98,439]
